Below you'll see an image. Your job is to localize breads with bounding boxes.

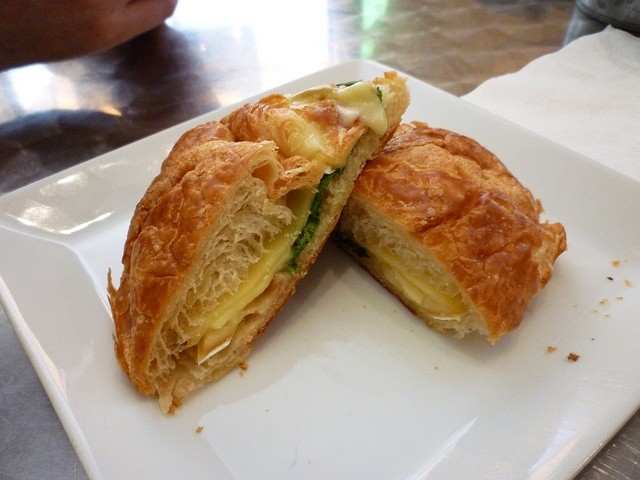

[336,119,566,345]
[104,71,413,408]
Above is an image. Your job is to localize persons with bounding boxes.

[0,0,182,73]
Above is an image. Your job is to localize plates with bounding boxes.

[1,59,640,479]
